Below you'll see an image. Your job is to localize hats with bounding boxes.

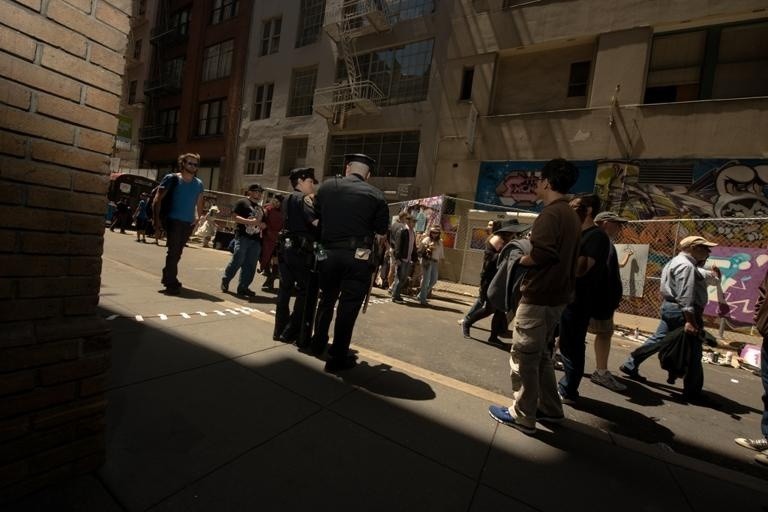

[249,184,265,192]
[595,210,629,224]
[678,235,719,248]
[343,151,377,170]
[288,166,319,185]
[406,215,419,221]
[494,218,533,236]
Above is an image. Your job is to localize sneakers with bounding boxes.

[536,407,566,422]
[734,438,767,466]
[375,282,429,307]
[237,287,256,297]
[161,279,184,295]
[200,244,211,249]
[274,332,327,354]
[488,405,537,435]
[457,317,473,340]
[619,364,647,382]
[221,279,229,294]
[590,369,617,393]
[323,356,356,373]
[487,338,508,348]
[256,267,280,293]
[108,224,159,246]
[556,389,580,405]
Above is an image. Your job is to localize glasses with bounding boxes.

[251,189,263,193]
[185,160,199,167]
[431,231,441,234]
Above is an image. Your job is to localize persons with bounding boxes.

[620,236,767,462]
[109,152,220,296]
[461,155,627,433]
[221,152,446,372]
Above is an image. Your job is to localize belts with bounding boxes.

[322,236,372,250]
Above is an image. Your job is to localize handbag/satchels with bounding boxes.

[146,172,179,222]
[276,192,307,273]
